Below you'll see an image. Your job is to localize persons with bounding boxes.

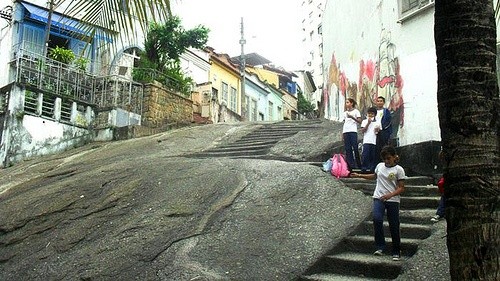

[340,96,391,173]
[350,147,406,260]
[431,177,445,222]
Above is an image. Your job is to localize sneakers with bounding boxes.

[393,254,401,261]
[430,214,440,222]
[375,250,385,256]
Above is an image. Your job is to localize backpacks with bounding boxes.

[332,153,351,178]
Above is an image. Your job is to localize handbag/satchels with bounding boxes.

[323,159,332,172]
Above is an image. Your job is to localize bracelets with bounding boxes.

[359,174,361,178]
